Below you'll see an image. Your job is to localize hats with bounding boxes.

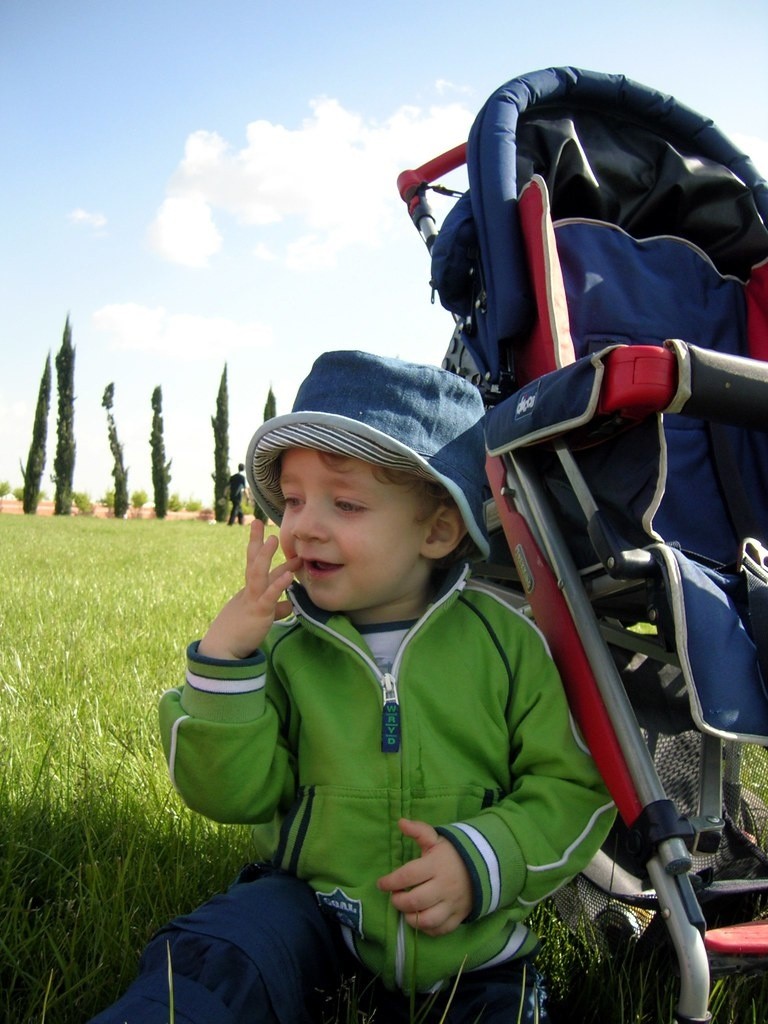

[246,350,492,565]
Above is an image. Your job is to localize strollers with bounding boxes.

[394,64,768,1024]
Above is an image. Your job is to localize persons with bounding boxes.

[222,464,250,526]
[90,345,620,1024]
